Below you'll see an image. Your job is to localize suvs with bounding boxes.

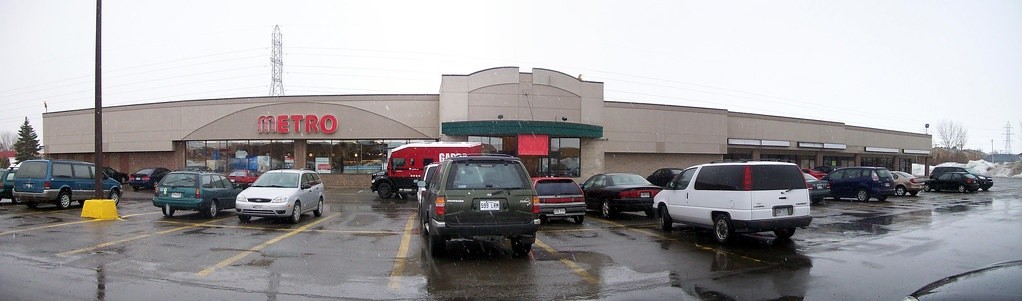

[412,154,541,259]
[152,166,325,225]
[930,167,994,191]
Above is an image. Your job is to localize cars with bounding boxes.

[922,171,980,193]
[580,174,663,220]
[530,177,587,225]
[802,165,831,204]
[647,167,684,188]
[103,166,129,185]
[545,163,571,176]
[890,170,927,197]
[126,167,171,191]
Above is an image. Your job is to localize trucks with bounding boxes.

[371,137,481,199]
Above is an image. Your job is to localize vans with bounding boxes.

[0,160,124,210]
[823,165,896,203]
[652,159,813,247]
